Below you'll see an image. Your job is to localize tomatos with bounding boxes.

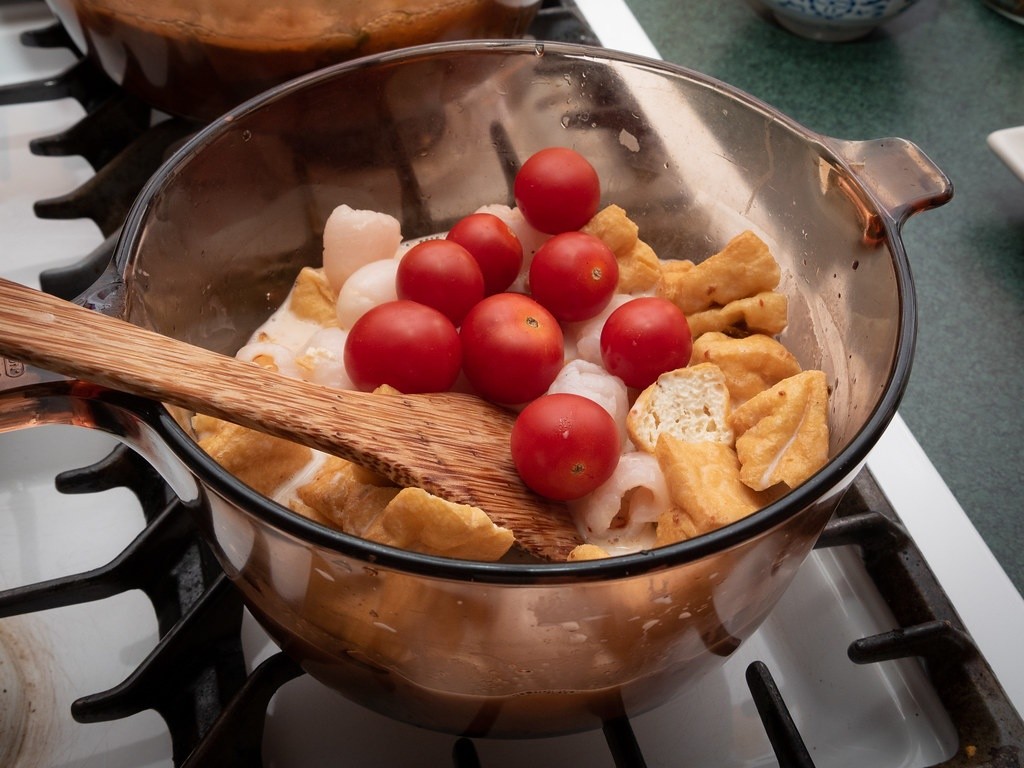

[515,145,600,233]
[343,213,694,500]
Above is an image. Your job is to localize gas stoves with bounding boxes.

[0,1,1024,768]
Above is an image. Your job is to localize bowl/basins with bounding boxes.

[761,0,916,42]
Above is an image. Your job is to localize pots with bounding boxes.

[1,37,953,733]
[42,1,541,125]
